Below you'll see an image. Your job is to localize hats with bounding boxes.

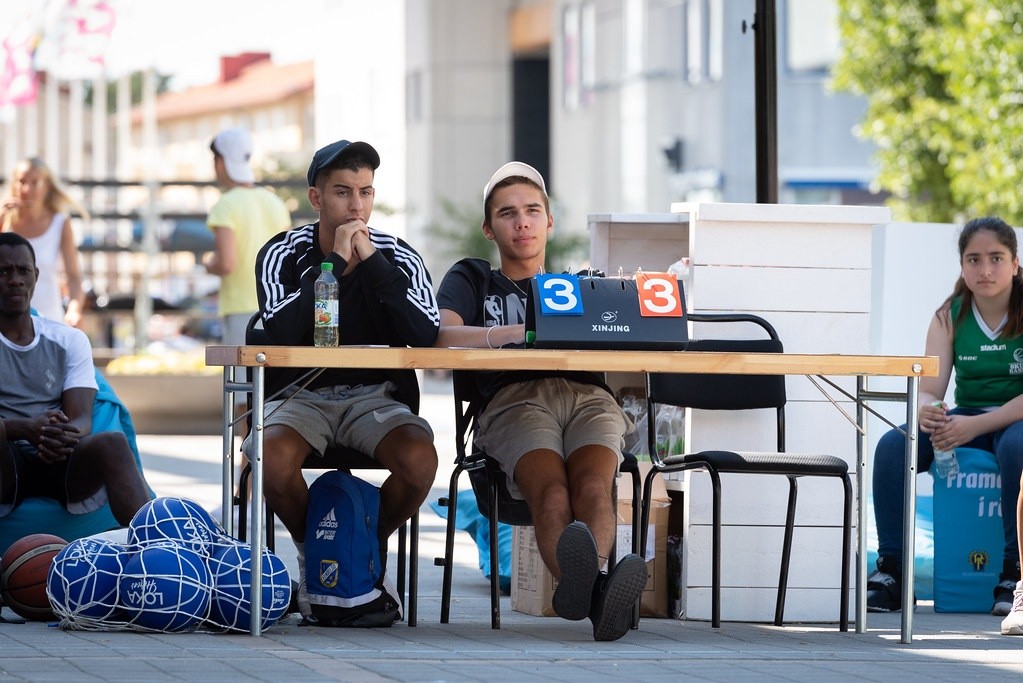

[308,140,381,188]
[482,162,549,214]
[214,127,257,183]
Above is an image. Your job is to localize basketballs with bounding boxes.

[0,533,70,622]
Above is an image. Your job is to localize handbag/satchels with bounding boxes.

[430,487,512,594]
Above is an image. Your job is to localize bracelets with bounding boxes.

[487,324,502,351]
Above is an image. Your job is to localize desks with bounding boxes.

[205,345,938,643]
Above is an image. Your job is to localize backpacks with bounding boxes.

[302,472,401,625]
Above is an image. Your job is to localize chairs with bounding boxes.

[238,311,852,630]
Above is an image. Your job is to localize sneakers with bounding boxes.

[991,560,1020,614]
[867,557,917,612]
[1001,581,1023,633]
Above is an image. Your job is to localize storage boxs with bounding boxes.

[510,462,672,620]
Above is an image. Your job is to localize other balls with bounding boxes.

[128,495,217,559]
[45,539,130,627]
[208,544,291,634]
[120,542,213,632]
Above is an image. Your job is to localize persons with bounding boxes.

[0,156,92,325]
[1001,470,1023,636]
[867,215,1023,614]
[202,126,292,528]
[0,232,152,620]
[436,161,650,642]
[244,140,441,616]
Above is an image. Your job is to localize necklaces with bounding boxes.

[500,271,527,295]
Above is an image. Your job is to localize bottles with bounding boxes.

[656,409,684,462]
[930,402,959,479]
[314,262,338,347]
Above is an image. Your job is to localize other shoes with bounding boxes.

[588,554,648,642]
[552,519,600,620]
[381,576,403,620]
[297,556,313,615]
